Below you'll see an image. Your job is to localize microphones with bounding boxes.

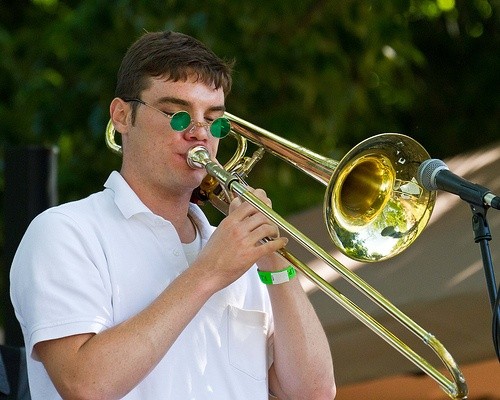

[416,159,500,210]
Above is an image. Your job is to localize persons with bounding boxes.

[10,33,340,400]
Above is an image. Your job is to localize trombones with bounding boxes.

[103,106,471,400]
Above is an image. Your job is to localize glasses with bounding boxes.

[124,98,231,138]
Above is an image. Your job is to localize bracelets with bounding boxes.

[257,266,297,286]
